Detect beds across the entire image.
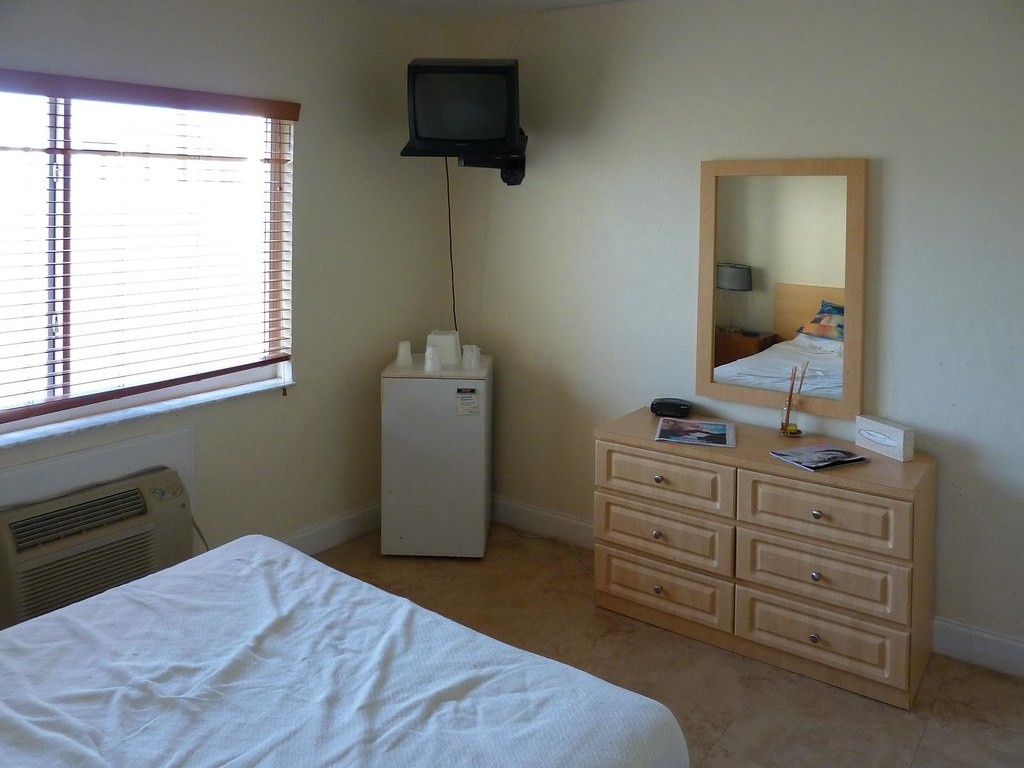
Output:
[0,534,694,768]
[714,282,845,401]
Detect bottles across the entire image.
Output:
[780,397,798,434]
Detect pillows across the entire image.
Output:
[789,333,844,353]
[796,299,844,342]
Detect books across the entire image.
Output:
[655,417,735,447]
[768,443,871,472]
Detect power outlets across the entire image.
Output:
[492,377,501,399]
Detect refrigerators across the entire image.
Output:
[378,353,494,558]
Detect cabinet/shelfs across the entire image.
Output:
[592,407,938,711]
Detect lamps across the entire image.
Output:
[717,263,753,332]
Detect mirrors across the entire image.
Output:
[695,158,869,419]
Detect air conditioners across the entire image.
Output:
[0,468,194,632]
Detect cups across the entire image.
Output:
[395,341,413,370]
[424,345,442,373]
[461,345,480,370]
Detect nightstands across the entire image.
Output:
[715,325,778,366]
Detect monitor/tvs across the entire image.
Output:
[407,57,519,153]
